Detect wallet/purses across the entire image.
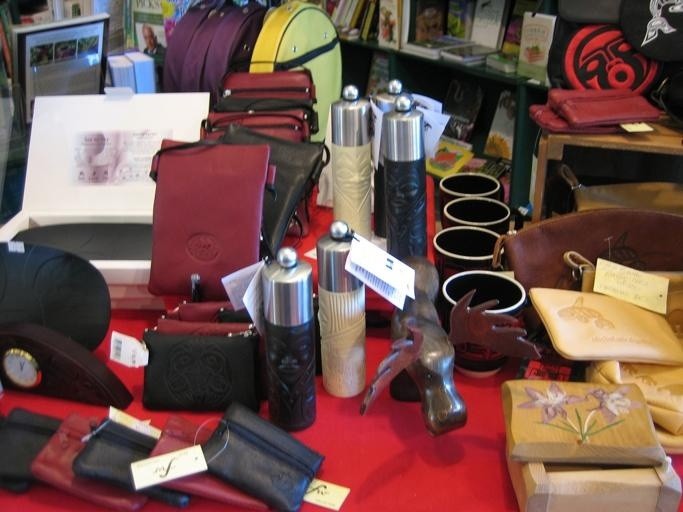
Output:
[30,413,148,510]
[142,304,264,414]
[0,405,63,493]
[0,404,322,511]
[72,415,191,508]
[202,404,324,512]
[528,90,662,136]
[148,416,270,511]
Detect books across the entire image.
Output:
[313,1,560,208]
[2,1,220,310]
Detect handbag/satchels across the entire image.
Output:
[528,287,683,368]
[501,379,666,466]
[492,210,683,296]
[564,251,683,336]
[585,361,683,434]
[145,70,326,297]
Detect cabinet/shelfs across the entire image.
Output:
[340,33,683,227]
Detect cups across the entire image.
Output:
[442,271,528,379]
[433,172,511,279]
